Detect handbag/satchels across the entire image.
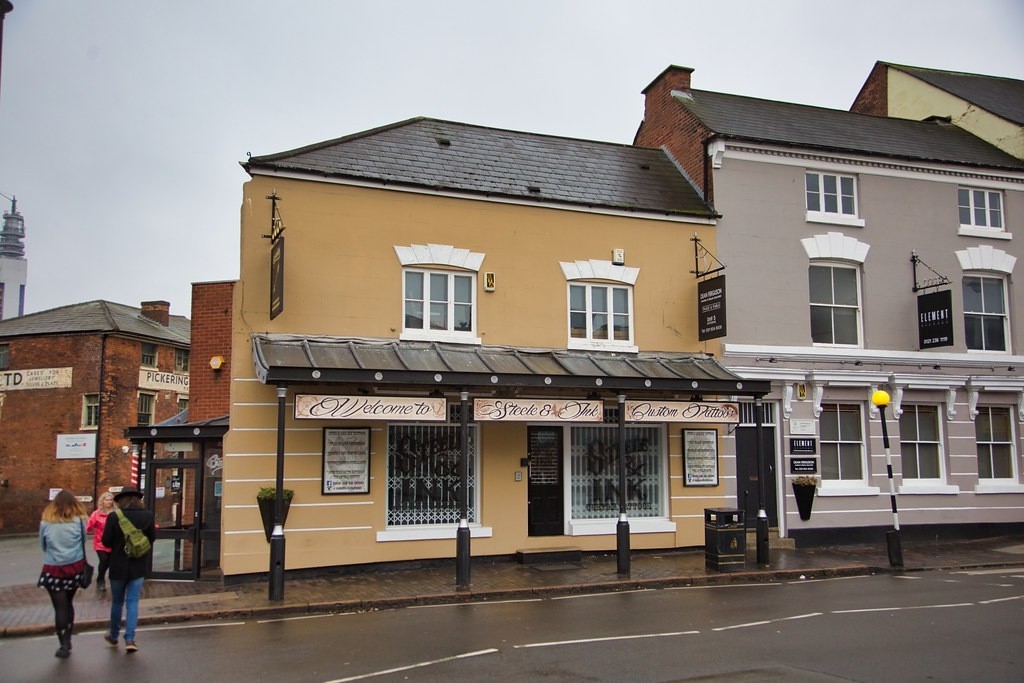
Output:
[79,562,94,588]
[116,509,152,558]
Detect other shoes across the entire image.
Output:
[97,579,106,591]
[56,648,68,657]
[67,640,71,649]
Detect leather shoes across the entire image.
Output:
[125,642,139,650]
[104,632,118,645]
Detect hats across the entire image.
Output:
[114,486,144,503]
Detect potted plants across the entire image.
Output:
[792,475,818,522]
[257,484,294,544]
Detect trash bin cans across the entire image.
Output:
[703,507,747,573]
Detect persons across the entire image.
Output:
[84,492,120,592]
[101,485,158,654]
[36,489,95,659]
